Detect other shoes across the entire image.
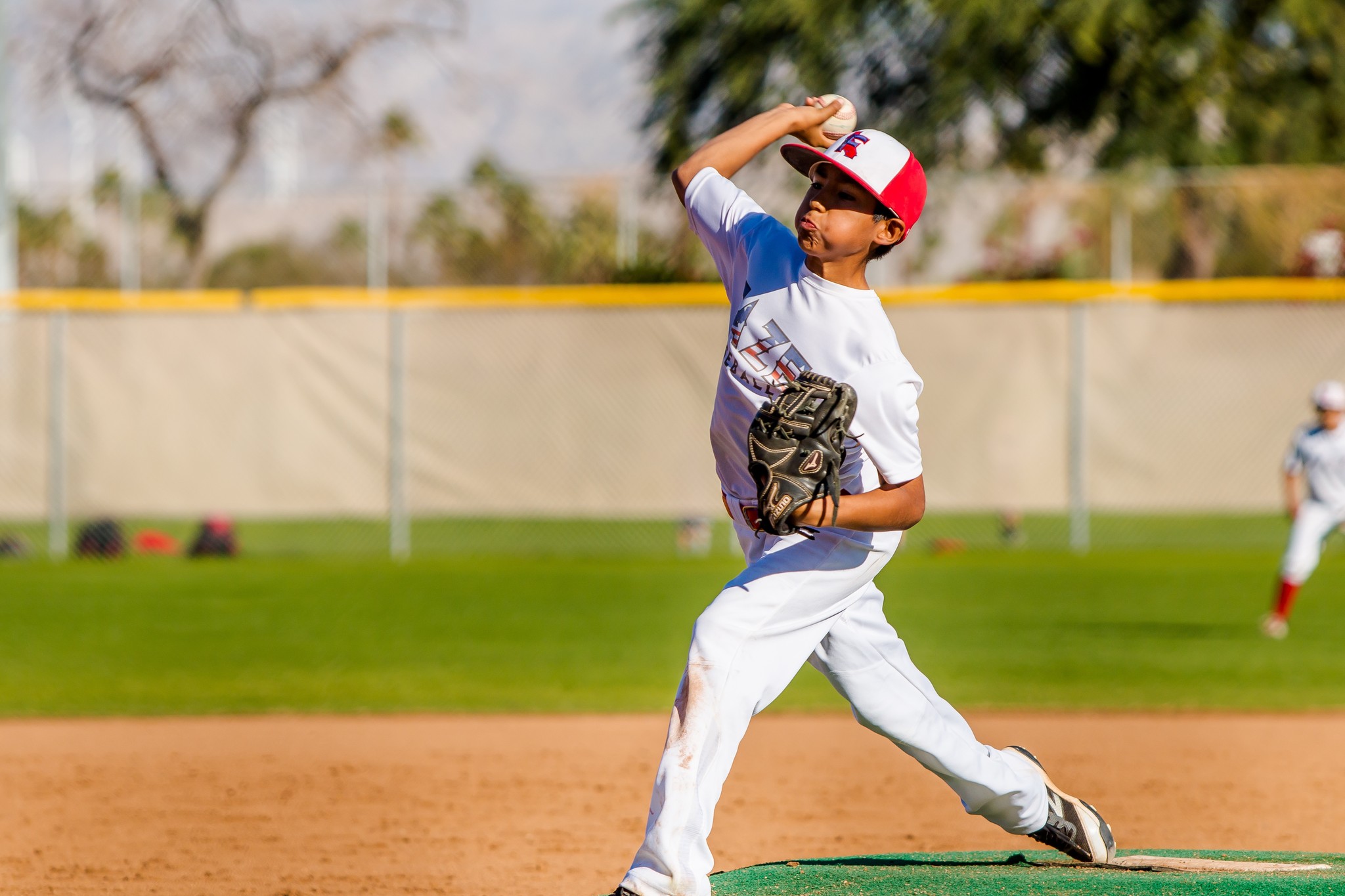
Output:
[1259,611,1291,641]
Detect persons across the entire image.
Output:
[1261,380,1345,640]
[607,99,1119,896]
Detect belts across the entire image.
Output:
[721,486,862,533]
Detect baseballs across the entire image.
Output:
[811,91,856,138]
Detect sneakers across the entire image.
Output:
[998,744,1117,865]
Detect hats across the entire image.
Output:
[778,128,928,246]
[1309,378,1345,413]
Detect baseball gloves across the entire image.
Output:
[747,369,857,540]
